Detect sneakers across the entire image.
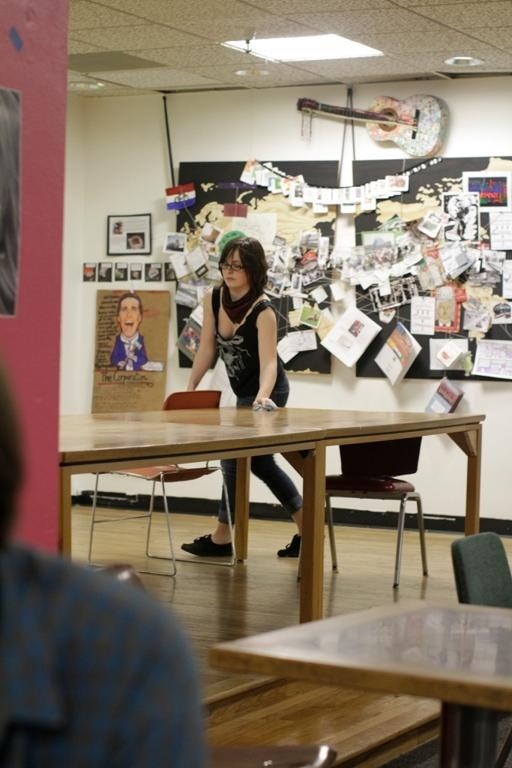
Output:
[278,533,301,558]
[182,535,233,557]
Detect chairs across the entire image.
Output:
[87,390,237,577]
[297,436,429,588]
[451,531,512,609]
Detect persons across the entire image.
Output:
[0,363,211,768]
[181,237,327,558]
[110,293,147,371]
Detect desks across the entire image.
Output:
[209,603,512,767]
[59,407,487,624]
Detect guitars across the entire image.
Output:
[298,95,449,159]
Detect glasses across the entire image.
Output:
[221,261,246,272]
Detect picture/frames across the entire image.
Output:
[463,169,511,212]
